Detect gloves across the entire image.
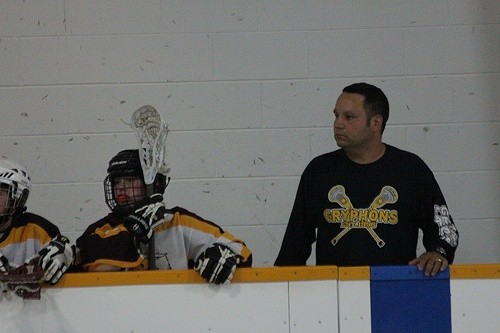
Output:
[194,243,240,285]
[26,235,75,284]
[0,253,10,275]
[126,192,174,243]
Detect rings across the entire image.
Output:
[436,259,442,263]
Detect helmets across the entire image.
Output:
[107,149,166,194]
[0,167,31,205]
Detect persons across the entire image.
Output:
[274,83,459,277]
[76,148,252,285]
[0,167,76,286]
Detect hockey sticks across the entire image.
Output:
[120,103,171,271]
